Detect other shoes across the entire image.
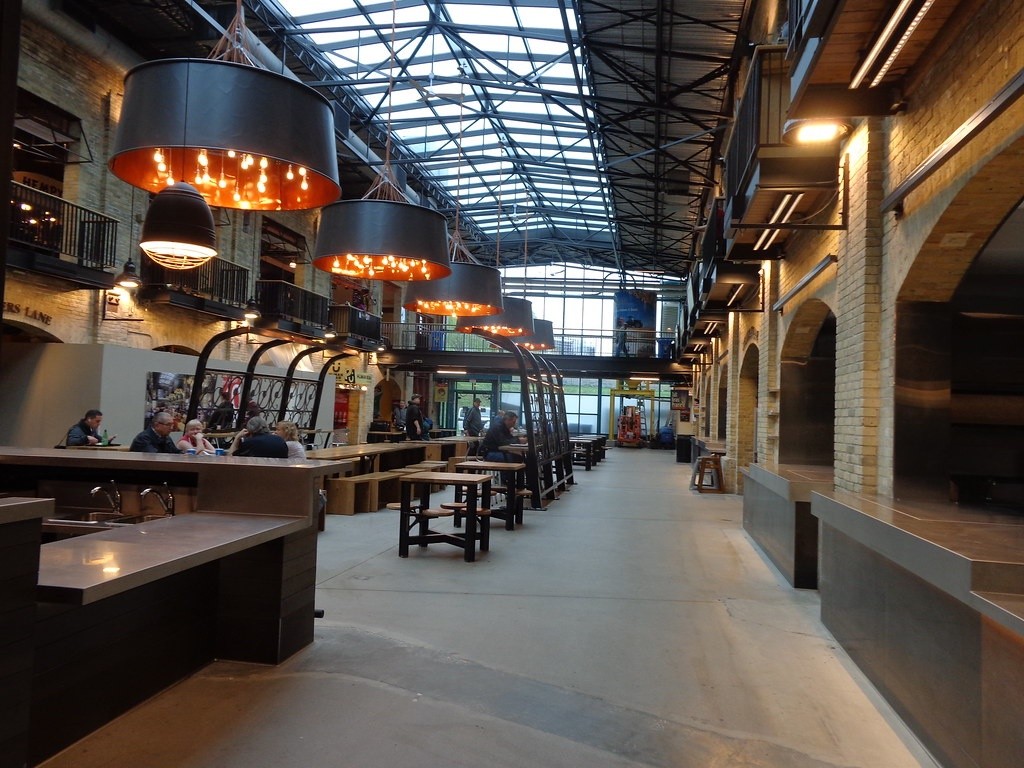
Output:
[515,487,532,496]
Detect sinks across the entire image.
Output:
[113,513,174,529]
[46,509,121,526]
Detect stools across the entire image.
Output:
[690,456,724,494]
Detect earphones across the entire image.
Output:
[88,418,90,421]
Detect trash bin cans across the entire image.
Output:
[676,435,695,463]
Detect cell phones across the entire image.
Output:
[112,435,116,438]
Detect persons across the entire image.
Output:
[406,394,433,441]
[275,419,307,459]
[463,398,483,462]
[352,287,369,310]
[207,391,234,430]
[392,400,407,432]
[65,408,112,446]
[175,418,216,454]
[227,416,289,459]
[480,407,527,491]
[245,401,261,428]
[129,411,184,454]
[615,322,630,357]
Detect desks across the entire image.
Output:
[203,428,607,563]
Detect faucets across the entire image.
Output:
[138,477,175,514]
[84,476,124,515]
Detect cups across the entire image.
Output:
[187,448,196,455]
[215,449,224,456]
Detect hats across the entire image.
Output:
[474,398,481,402]
[412,394,422,400]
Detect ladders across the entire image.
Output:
[637,398,648,443]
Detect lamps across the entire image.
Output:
[455,141,535,338]
[240,211,262,319]
[107,0,341,212]
[114,185,143,288]
[403,84,504,317]
[313,0,452,282]
[323,276,339,338]
[782,118,855,146]
[377,301,387,350]
[489,187,555,351]
[138,0,219,270]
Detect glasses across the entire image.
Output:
[157,421,174,427]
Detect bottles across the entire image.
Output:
[102,429,108,446]
